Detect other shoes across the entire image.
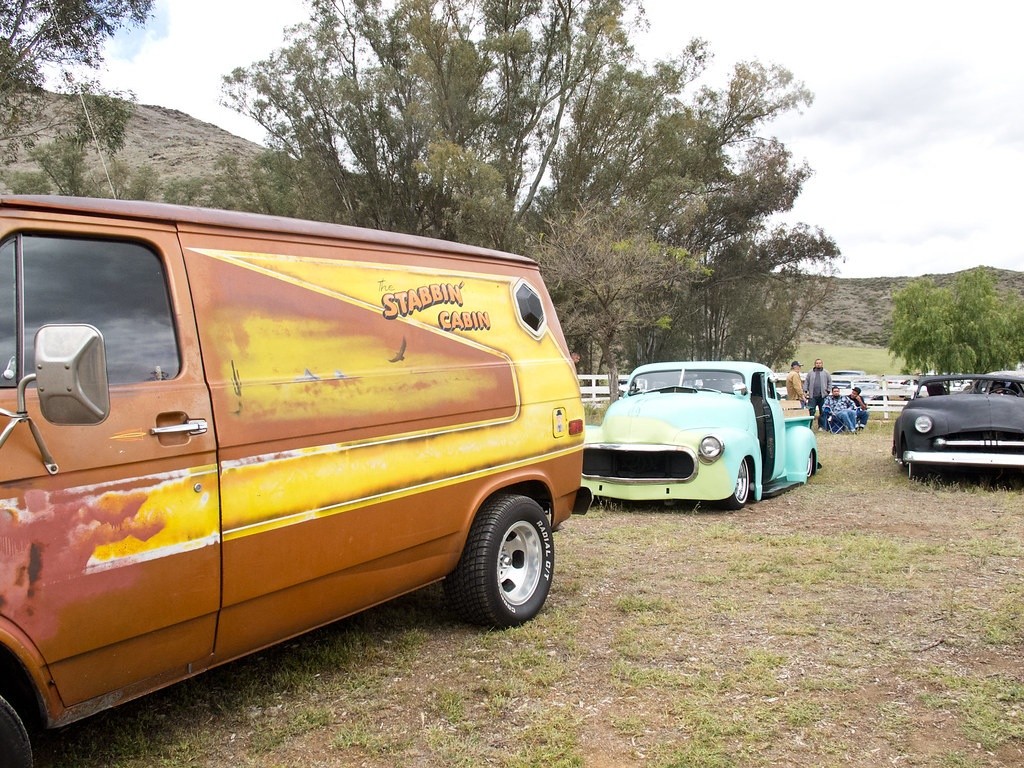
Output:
[817,428,824,432]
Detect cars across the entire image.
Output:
[580,360,822,512]
[829,370,866,389]
[857,385,889,407]
[892,374,1024,481]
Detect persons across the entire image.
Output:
[571,352,580,365]
[786,361,806,405]
[848,387,869,430]
[803,359,831,431]
[822,387,857,433]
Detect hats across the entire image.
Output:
[852,387,862,394]
[791,361,803,367]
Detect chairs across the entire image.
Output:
[703,380,733,393]
[824,407,848,434]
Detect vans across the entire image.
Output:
[0,194,593,768]
